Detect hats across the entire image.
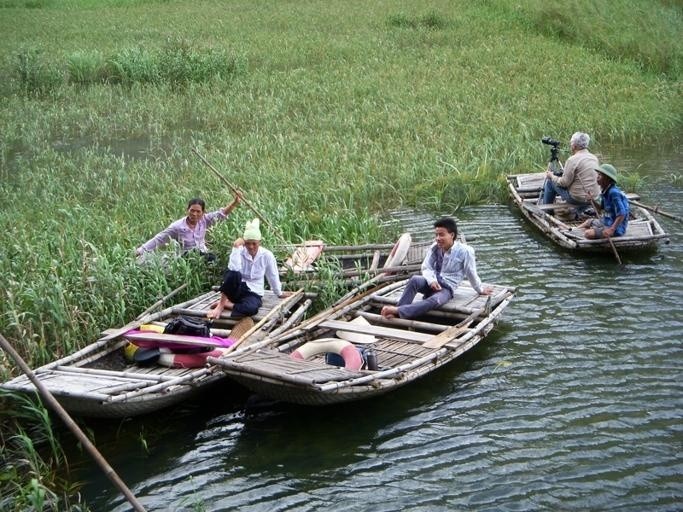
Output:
[242,219,261,241]
[335,314,380,343]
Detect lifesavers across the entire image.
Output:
[289,338,364,371]
[124,325,165,365]
[383,233,412,276]
[157,346,228,368]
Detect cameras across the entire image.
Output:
[540,135,563,151]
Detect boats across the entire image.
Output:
[207,275,517,403]
[1,279,311,424]
[504,170,668,255]
[275,235,468,286]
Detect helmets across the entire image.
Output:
[594,164,618,184]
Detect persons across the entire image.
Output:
[381,217,492,321]
[129,189,241,264]
[542,130,600,217]
[577,165,629,240]
[205,218,289,320]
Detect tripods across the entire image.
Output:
[535,156,565,206]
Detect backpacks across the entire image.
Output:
[162,315,215,353]
[325,344,370,370]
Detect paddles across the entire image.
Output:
[205,241,328,247]
[422,292,514,348]
[98,283,187,340]
[522,202,572,231]
[301,271,386,330]
